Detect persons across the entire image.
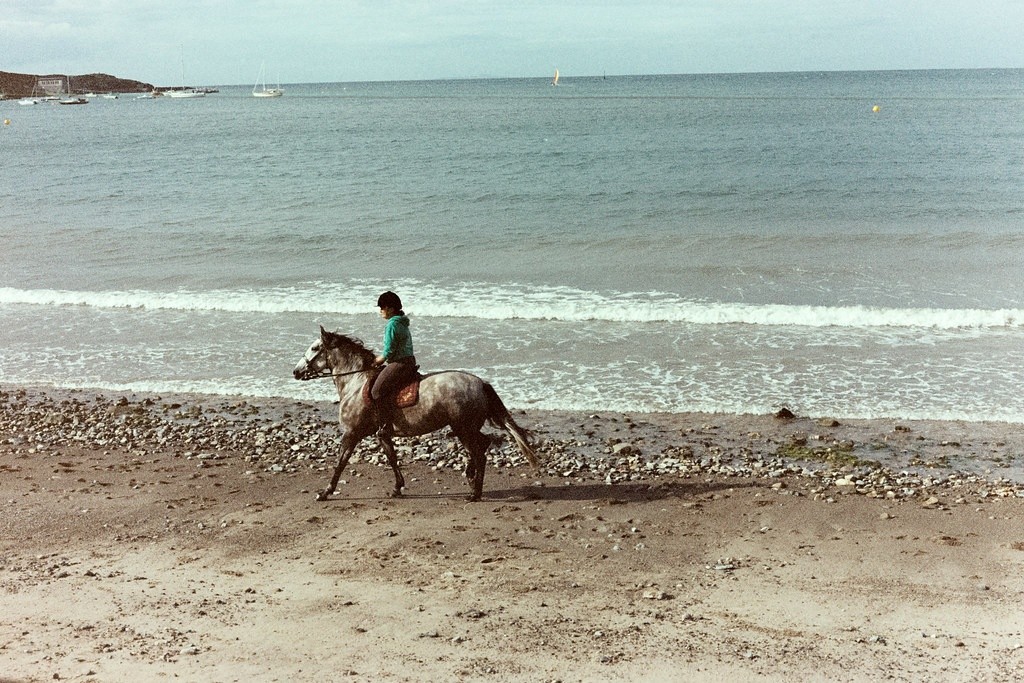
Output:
[368,290,416,436]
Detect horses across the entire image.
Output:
[292,326,540,501]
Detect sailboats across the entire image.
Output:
[251,60,286,99]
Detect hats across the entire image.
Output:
[373,291,402,310]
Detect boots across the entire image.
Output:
[373,395,395,437]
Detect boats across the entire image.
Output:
[104,94,118,100]
[59,98,91,105]
[138,86,221,97]
[18,95,61,107]
